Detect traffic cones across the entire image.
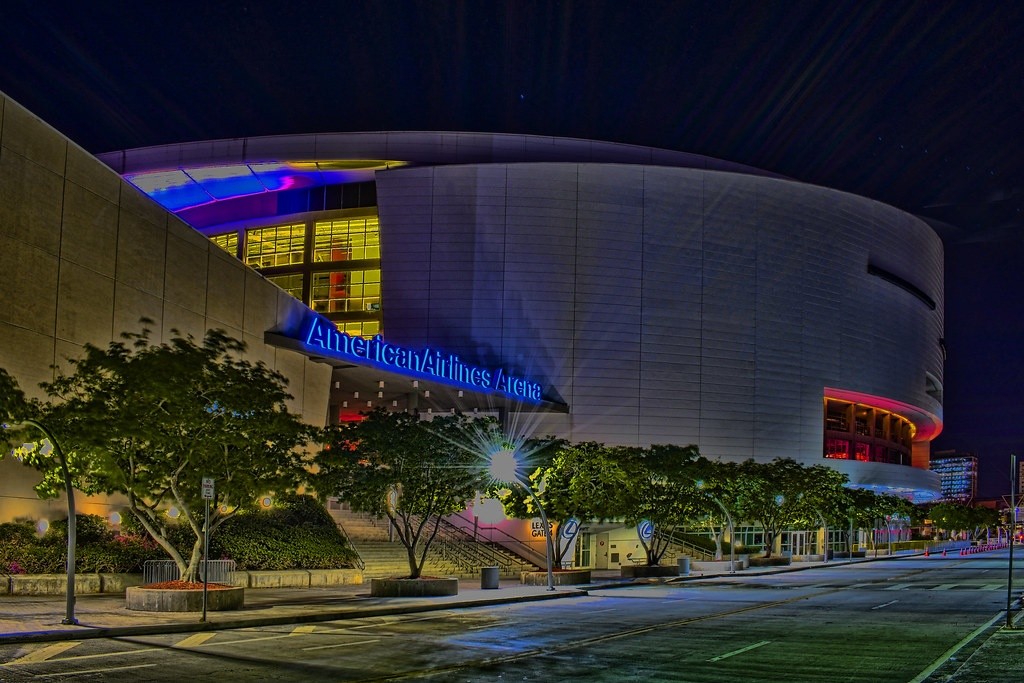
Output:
[925,546,930,556]
[960,542,1007,555]
[942,548,946,556]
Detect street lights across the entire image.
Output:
[0,419,78,624]
[800,493,828,563]
[492,458,555,591]
[698,482,736,573]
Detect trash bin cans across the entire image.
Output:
[827,550,833,559]
[677,559,689,576]
[739,554,750,567]
[860,548,866,555]
[893,543,899,551]
[481,568,499,589]
[782,551,792,562]
